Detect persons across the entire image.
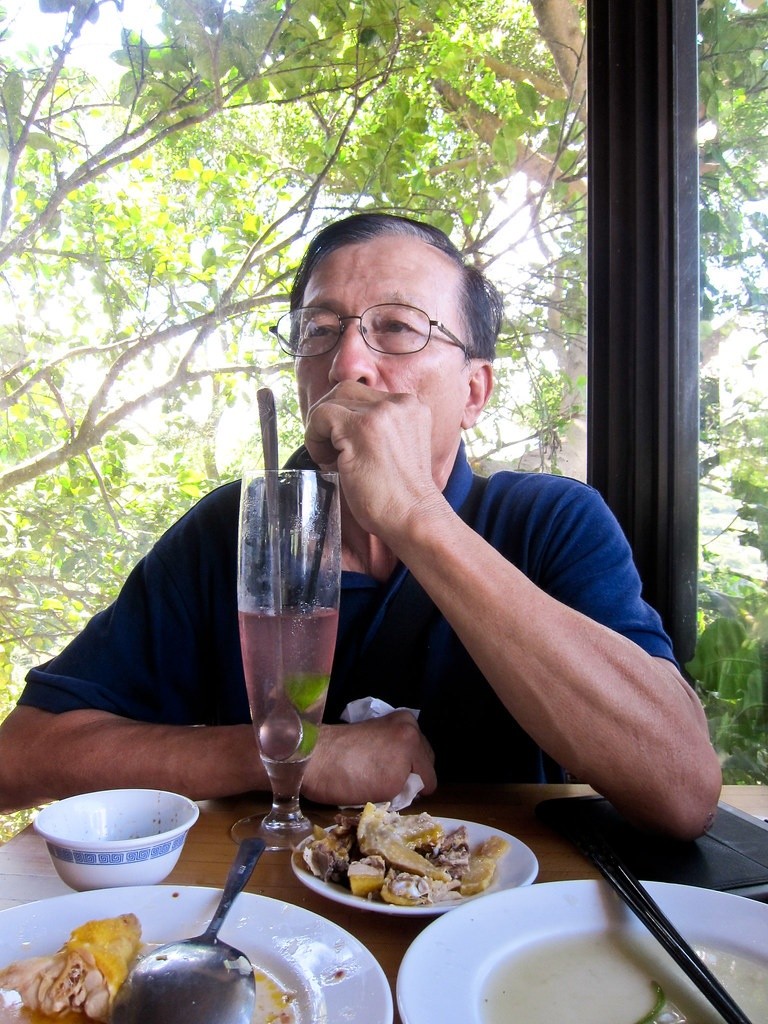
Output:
[0,206,721,843]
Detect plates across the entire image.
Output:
[289,810,541,916]
[2,883,392,1024]
[394,872,768,1023]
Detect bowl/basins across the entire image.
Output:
[32,788,199,895]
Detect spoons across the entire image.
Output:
[254,382,307,761]
[109,836,261,1024]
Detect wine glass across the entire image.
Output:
[230,466,343,850]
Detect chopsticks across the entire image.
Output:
[584,838,752,1023]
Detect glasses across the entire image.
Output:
[267,303,469,362]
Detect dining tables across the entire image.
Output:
[0,800,768,1024]
